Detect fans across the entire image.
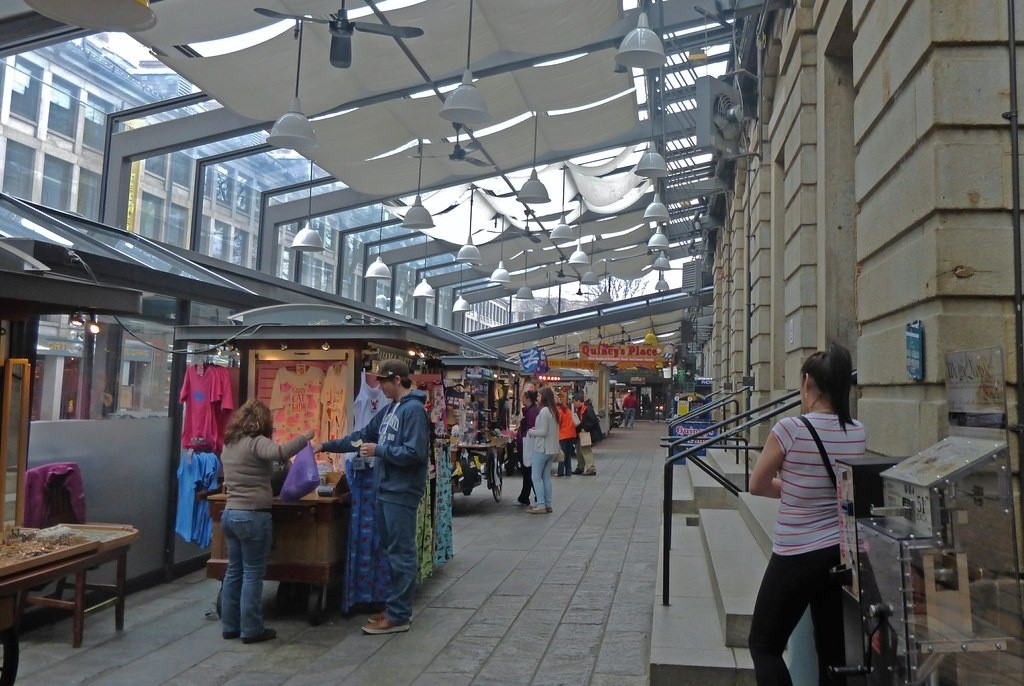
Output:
[252,0,426,70]
[487,209,551,243]
[408,123,494,168]
[695,75,743,156]
[551,257,577,280]
[569,273,591,296]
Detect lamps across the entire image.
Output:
[438,0,492,126]
[292,160,325,253]
[516,110,551,204]
[412,236,435,299]
[401,140,436,228]
[407,345,425,358]
[568,198,591,266]
[452,265,470,313]
[489,215,511,284]
[516,249,534,300]
[364,206,392,281]
[265,14,318,149]
[457,187,483,261]
[614,0,671,290]
[538,279,557,316]
[19,0,158,35]
[583,237,598,287]
[548,168,576,242]
[66,309,102,336]
[596,259,613,304]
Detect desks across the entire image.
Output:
[204,491,352,627]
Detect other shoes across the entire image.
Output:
[571,469,584,474]
[242,628,277,644]
[564,473,570,476]
[513,499,526,506]
[547,507,553,513]
[222,631,239,639]
[582,470,596,476]
[527,507,546,513]
[555,474,564,476]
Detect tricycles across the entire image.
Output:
[437,356,520,502]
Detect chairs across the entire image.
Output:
[24,460,131,649]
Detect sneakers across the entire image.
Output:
[367,611,412,625]
[361,616,410,633]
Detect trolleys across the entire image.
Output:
[196,471,353,626]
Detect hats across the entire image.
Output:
[628,389,632,392]
[366,359,409,378]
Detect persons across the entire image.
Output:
[517,390,539,505]
[622,391,639,429]
[555,395,577,477]
[748,341,866,686]
[222,398,314,644]
[572,395,603,476]
[526,387,559,514]
[642,394,650,415]
[311,359,430,633]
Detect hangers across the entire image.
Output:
[186,446,212,455]
[191,353,236,369]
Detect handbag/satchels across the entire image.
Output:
[579,429,591,446]
[557,445,565,462]
[572,413,581,427]
[522,430,534,466]
[280,440,321,502]
